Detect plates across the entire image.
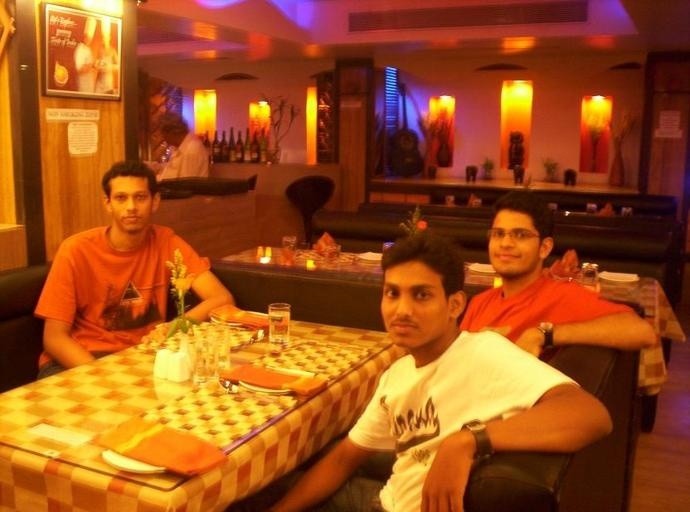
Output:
[356,249,385,263]
[599,269,641,285]
[467,261,495,274]
[211,310,269,325]
[239,363,316,393]
[102,448,167,475]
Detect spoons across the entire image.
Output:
[219,377,281,398]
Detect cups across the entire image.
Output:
[471,198,482,207]
[622,206,632,216]
[323,242,342,269]
[445,195,455,205]
[586,204,596,214]
[282,235,297,263]
[269,302,290,345]
[382,242,394,252]
[185,324,232,384]
[549,203,558,212]
[580,262,599,287]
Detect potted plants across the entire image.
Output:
[609,110,635,186]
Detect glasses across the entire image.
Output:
[487,228,541,243]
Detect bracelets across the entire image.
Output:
[458,418,494,460]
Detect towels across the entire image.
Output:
[88,414,228,477]
[218,363,328,399]
[209,303,290,333]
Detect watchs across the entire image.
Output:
[536,319,555,351]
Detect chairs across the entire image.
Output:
[283,176,335,246]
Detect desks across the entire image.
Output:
[224,244,687,433]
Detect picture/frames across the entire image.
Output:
[41,1,124,103]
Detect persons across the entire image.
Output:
[455,193,658,362]
[242,234,615,512]
[155,111,211,183]
[35,159,236,387]
[395,125,424,178]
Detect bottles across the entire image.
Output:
[207,127,267,163]
[152,348,191,384]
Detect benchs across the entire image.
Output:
[464,299,645,512]
[0,260,53,392]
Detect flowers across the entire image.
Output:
[397,204,431,250]
[163,248,201,338]
